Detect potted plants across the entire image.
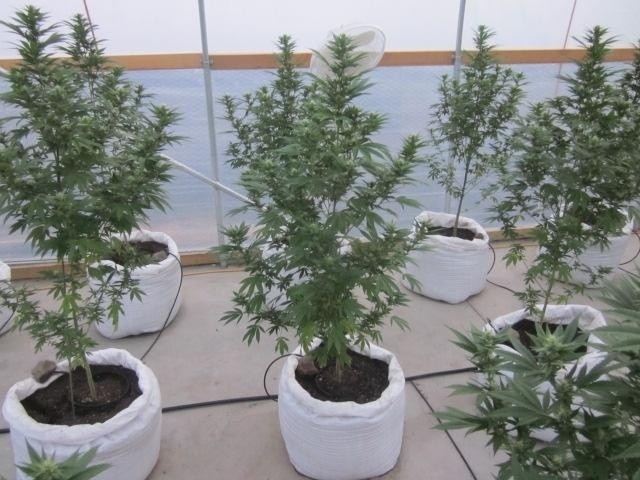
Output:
[209,29,447,479]
[13,437,111,479]
[545,28,638,289]
[258,32,354,322]
[0,3,163,480]
[85,70,183,338]
[476,25,640,447]
[401,25,532,306]
[433,268,639,479]
